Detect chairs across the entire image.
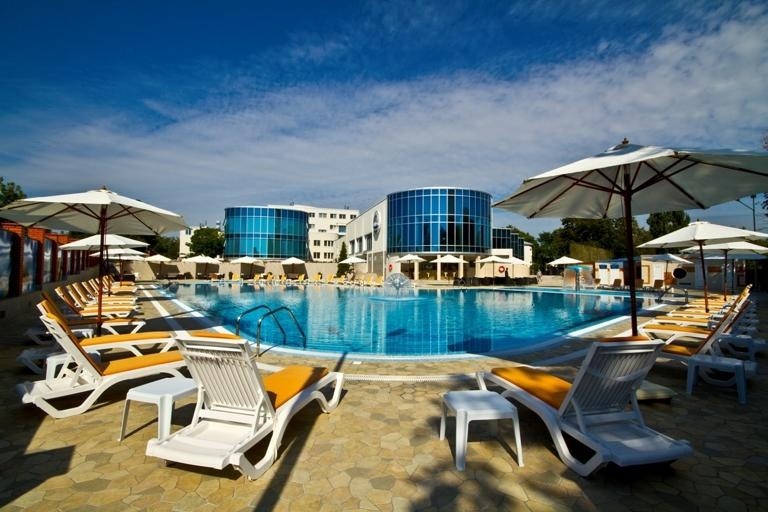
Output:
[13,275,197,419]
[146,331,345,481]
[599,283,767,405]
[413,269,455,280]
[454,276,536,286]
[585,278,664,293]
[165,271,383,287]
[476,336,694,477]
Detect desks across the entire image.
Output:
[439,390,525,472]
[117,377,201,466]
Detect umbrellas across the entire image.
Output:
[3,187,187,337]
[490,138,768,337]
[635,219,766,314]
[679,239,766,302]
